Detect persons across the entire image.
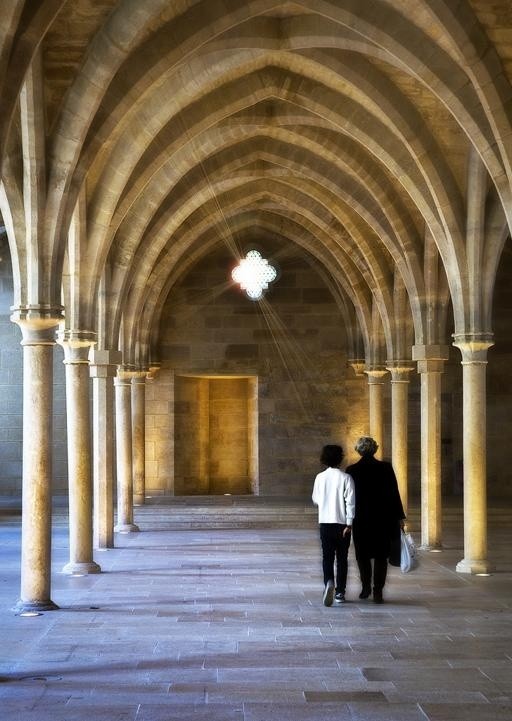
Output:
[343,436,407,606]
[310,443,358,608]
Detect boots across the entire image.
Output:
[358,574,373,600]
[373,586,386,604]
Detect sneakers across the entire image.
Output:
[320,579,335,608]
[335,593,346,603]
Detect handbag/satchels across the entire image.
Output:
[388,527,420,575]
